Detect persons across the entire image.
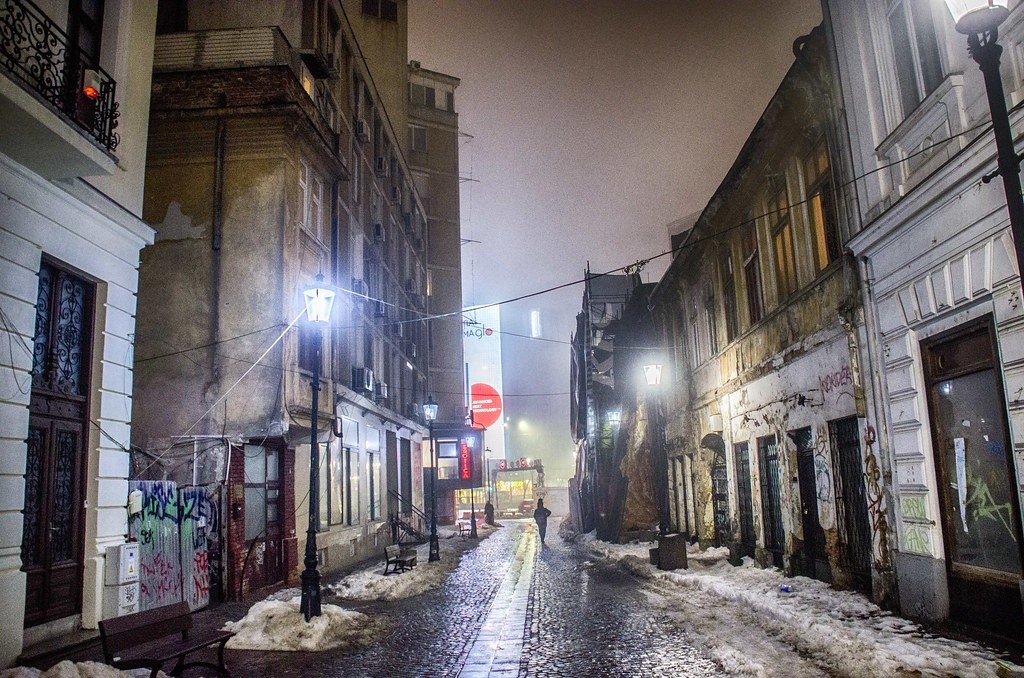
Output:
[484,501,495,526]
[534,498,552,543]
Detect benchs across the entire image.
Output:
[459,523,470,537]
[384,544,416,576]
[98,600,237,678]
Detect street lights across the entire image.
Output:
[485,447,491,502]
[642,359,669,535]
[943,0,1024,295]
[299,273,338,624]
[422,394,441,563]
[464,434,478,538]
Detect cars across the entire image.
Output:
[519,499,534,512]
